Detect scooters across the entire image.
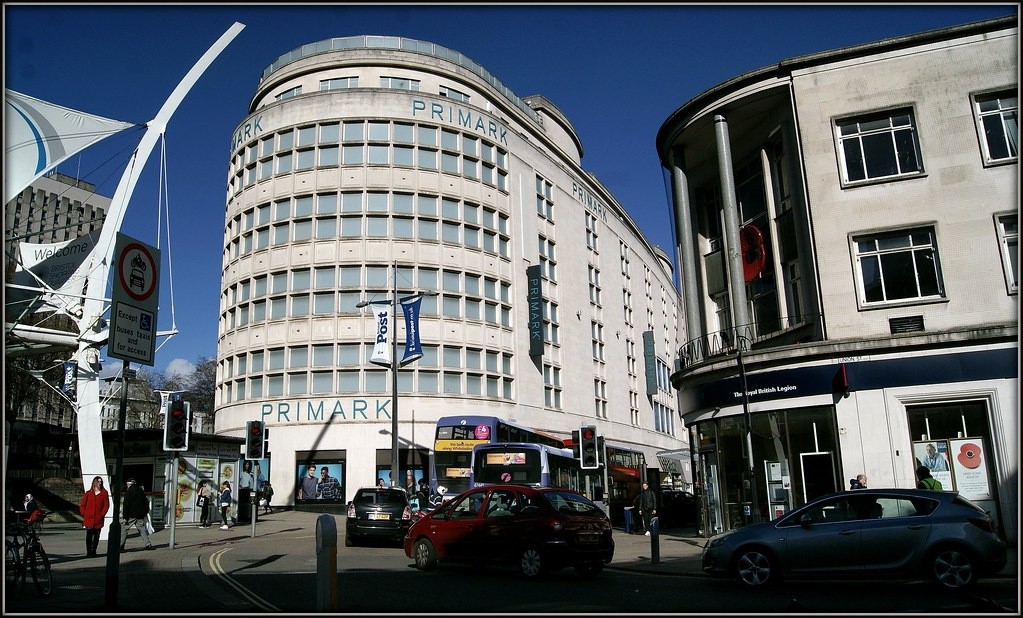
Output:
[407,503,466,529]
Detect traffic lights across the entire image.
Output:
[245,420,266,461]
[572,430,580,458]
[163,399,190,452]
[580,425,599,469]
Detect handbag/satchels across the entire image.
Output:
[408,494,420,512]
[196,488,206,507]
[259,498,267,507]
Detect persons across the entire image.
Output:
[639,481,656,537]
[218,480,232,530]
[916,467,942,490]
[80,476,109,558]
[403,470,416,494]
[196,479,211,528]
[298,464,321,500]
[316,467,341,499]
[120,478,151,551]
[923,444,946,472]
[24,494,38,520]
[378,478,384,487]
[850,474,867,490]
[239,459,265,487]
[262,482,274,515]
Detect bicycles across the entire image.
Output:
[5,506,56,598]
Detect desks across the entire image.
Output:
[12,510,29,533]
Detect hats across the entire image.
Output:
[126,478,137,482]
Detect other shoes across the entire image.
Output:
[645,531,650,536]
[86,551,94,558]
[92,552,99,558]
[119,544,125,552]
[198,523,210,528]
[269,512,273,514]
[219,524,228,530]
[145,544,153,550]
[263,512,267,515]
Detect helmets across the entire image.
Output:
[436,486,448,496]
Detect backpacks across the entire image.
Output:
[264,484,274,498]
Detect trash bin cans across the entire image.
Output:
[237,489,259,521]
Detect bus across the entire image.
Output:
[428,415,563,511]
[470,442,616,521]
[563,439,648,521]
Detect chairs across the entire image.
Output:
[26,510,52,536]
[487,495,510,516]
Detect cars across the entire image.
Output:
[699,488,1007,595]
[662,490,696,524]
[403,484,615,582]
[345,487,418,549]
